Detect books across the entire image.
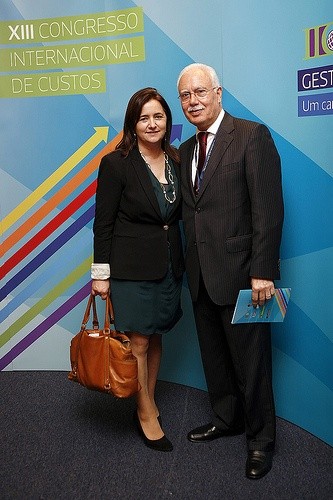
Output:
[232,288,292,324]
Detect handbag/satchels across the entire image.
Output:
[67,292,141,399]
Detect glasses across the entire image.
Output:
[177,86,220,104]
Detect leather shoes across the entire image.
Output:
[187,422,246,442]
[246,447,275,480]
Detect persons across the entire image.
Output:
[175,63,284,481]
[91,87,183,452]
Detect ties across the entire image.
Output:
[193,132,209,198]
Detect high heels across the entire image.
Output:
[156,411,163,426]
[134,409,173,452]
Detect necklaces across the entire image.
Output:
[138,150,176,204]
